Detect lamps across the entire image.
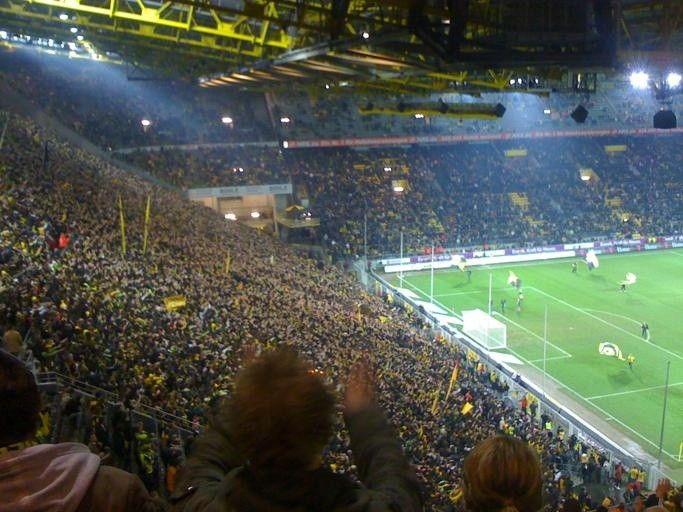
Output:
[510,74,541,89]
[573,72,589,91]
[613,71,681,92]
[448,80,468,89]
